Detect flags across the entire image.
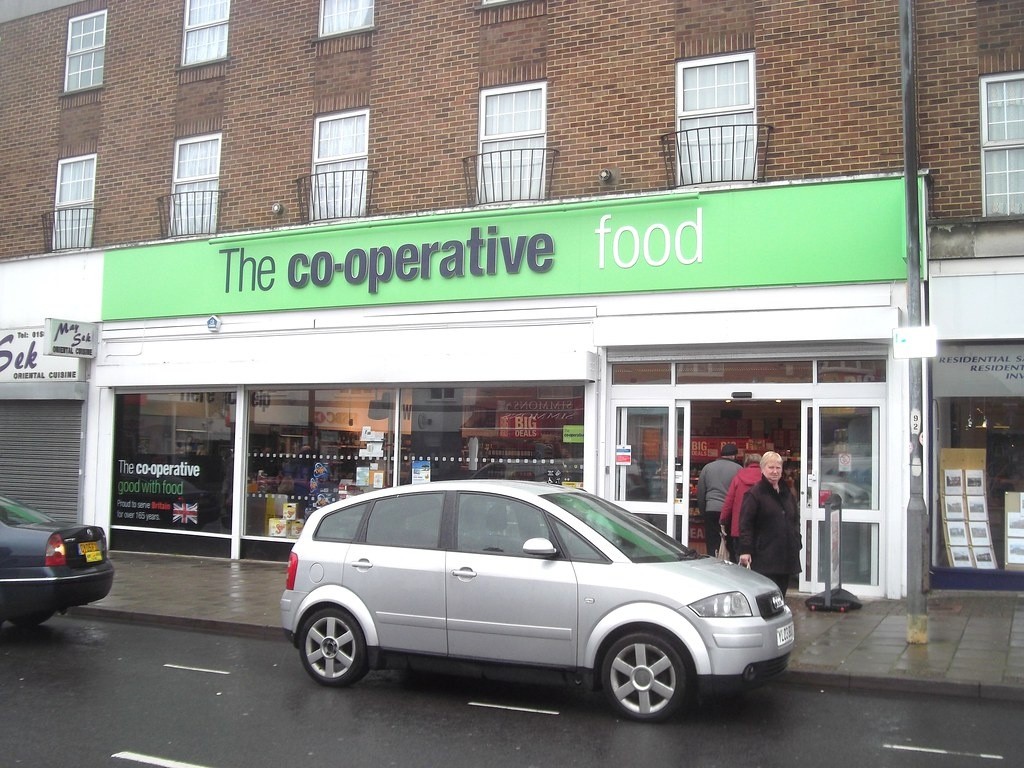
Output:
[173,502,198,525]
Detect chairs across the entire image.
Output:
[459,497,518,555]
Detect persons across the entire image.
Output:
[719,452,763,566]
[783,460,793,478]
[220,450,294,515]
[618,457,653,547]
[181,435,208,456]
[697,443,743,558]
[301,443,318,464]
[736,450,802,600]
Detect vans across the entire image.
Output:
[471,460,560,482]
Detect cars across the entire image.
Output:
[0,496,115,627]
[118,470,222,531]
[280,479,795,723]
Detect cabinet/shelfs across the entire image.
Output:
[644,474,706,543]
[174,428,213,453]
[320,446,411,464]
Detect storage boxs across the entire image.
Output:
[268,503,305,537]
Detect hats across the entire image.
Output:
[720,444,738,456]
